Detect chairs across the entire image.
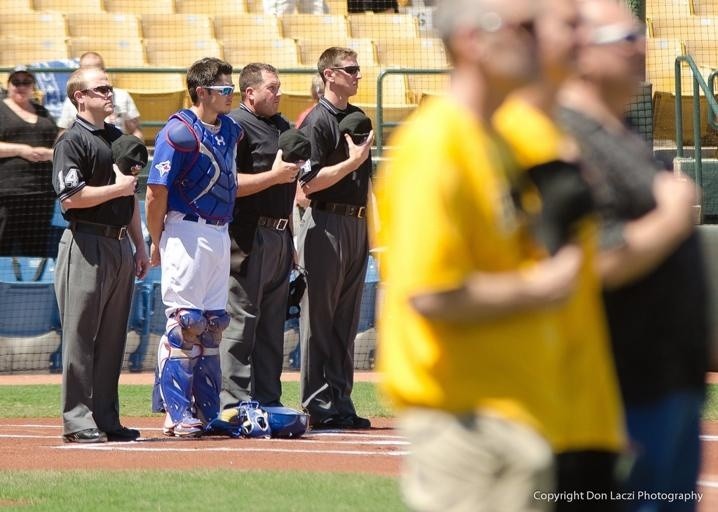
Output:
[645,0,718,146]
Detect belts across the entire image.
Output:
[258,216,289,232]
[309,201,367,219]
[183,215,226,227]
[68,221,129,242]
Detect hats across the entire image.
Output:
[513,158,592,259]
[338,111,373,157]
[111,134,148,177]
[8,65,37,84]
[278,128,310,165]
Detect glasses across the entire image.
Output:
[72,86,113,101]
[331,65,360,75]
[11,79,32,86]
[195,84,235,97]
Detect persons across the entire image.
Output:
[298,46,379,430]
[144,55,244,440]
[220,61,306,410]
[289,70,325,212]
[0,64,57,257]
[263,1,400,16]
[52,66,151,445]
[58,51,141,138]
[366,1,712,512]
